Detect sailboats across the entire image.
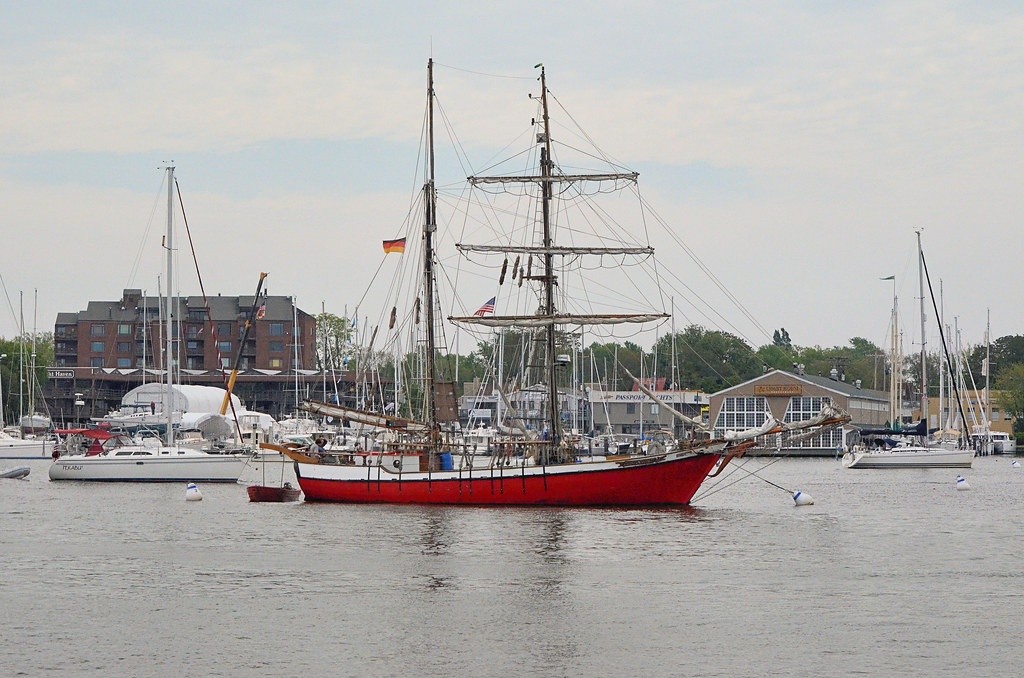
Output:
[259,58,853,507]
[840,229,992,469]
[0,160,267,482]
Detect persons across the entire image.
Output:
[310,438,327,459]
[386,438,399,452]
[150,401,155,415]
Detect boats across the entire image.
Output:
[242,447,303,502]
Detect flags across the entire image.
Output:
[473,296,495,317]
[383,237,406,254]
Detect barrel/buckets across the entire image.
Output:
[441,451,452,470]
